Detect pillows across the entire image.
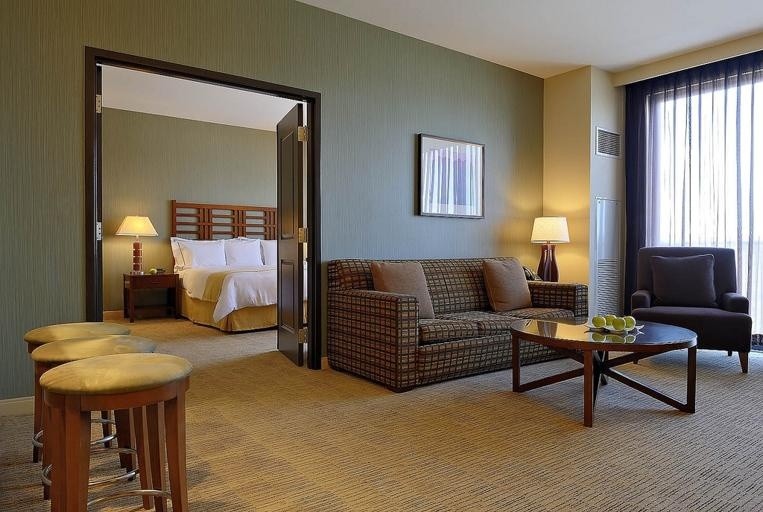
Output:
[482,259,533,313]
[171,236,277,274]
[369,262,436,319]
[649,253,720,308]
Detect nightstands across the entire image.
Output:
[122,272,179,323]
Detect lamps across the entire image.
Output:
[113,215,159,275]
[529,217,570,282]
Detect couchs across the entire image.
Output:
[326,256,589,393]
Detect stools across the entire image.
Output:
[22,320,194,511]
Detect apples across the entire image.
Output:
[592,333,636,344]
[149,269,158,275]
[591,314,636,330]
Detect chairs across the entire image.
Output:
[630,246,752,374]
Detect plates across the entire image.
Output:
[583,320,643,335]
[582,330,645,344]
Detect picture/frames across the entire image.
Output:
[414,133,485,220]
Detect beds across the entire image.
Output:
[170,200,307,335]
